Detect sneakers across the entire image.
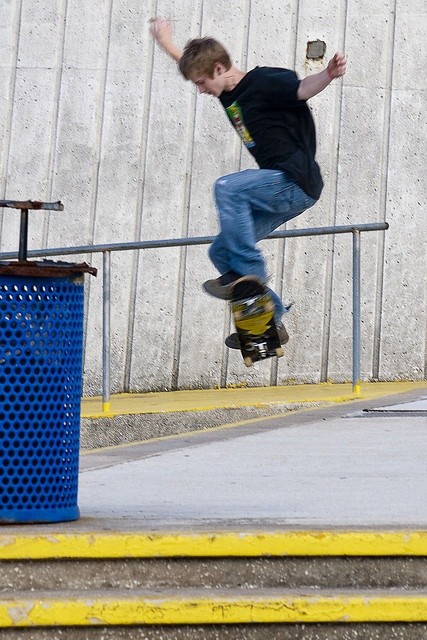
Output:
[225,323,289,348]
[202,270,264,300]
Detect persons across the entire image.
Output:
[138,15,347,350]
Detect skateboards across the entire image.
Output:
[229,274,283,367]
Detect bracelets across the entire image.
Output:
[325,67,336,81]
